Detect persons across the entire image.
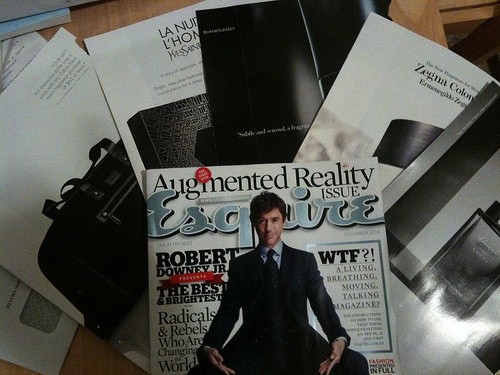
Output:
[185,190,369,375]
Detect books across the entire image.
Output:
[145,156,402,375]
[83,0,220,208]
[1,27,148,343]
[195,0,394,165]
[383,82,500,375]
[291,12,500,191]
[1,0,100,42]
